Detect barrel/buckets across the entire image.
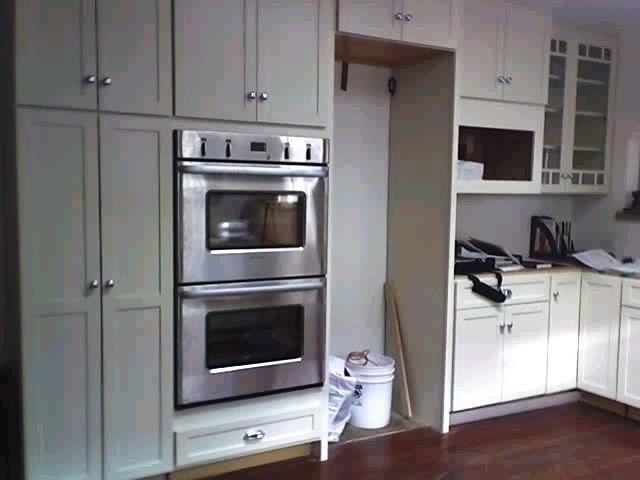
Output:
[345,357,396,429]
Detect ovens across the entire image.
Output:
[173,129,326,405]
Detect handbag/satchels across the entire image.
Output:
[455,255,505,303]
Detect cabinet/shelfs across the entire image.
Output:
[449,271,640,411]
[336,2,459,67]
[8,105,169,480]
[542,24,616,193]
[9,0,335,127]
[388,8,549,105]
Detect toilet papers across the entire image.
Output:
[624,139,640,193]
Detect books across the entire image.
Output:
[538,218,573,256]
